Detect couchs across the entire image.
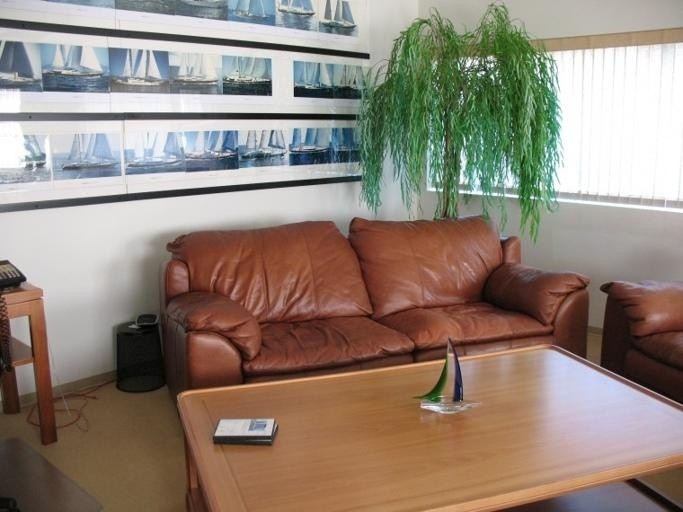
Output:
[160,235,590,418]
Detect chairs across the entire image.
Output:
[599,279,683,407]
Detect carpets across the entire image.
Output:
[0,439,105,512]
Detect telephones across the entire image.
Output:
[0,260,26,288]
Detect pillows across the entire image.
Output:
[169,220,373,325]
[349,215,504,319]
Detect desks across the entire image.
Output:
[0,282,57,445]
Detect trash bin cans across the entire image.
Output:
[116,321,165,392]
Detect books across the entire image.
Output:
[213,418,275,441]
[213,424,278,446]
